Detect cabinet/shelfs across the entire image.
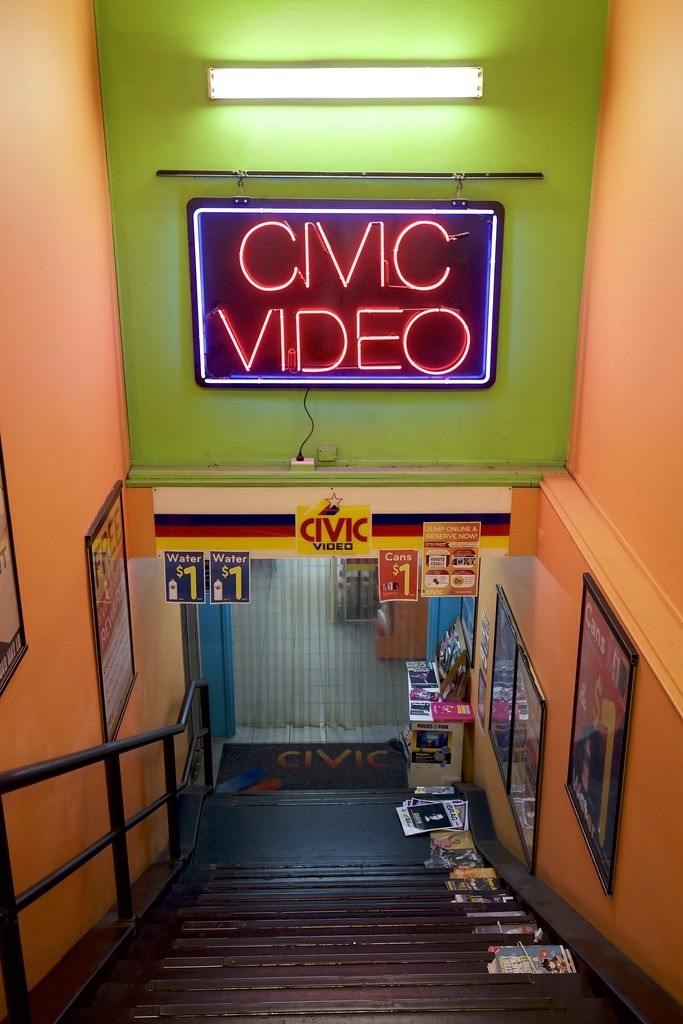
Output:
[375,566,428,659]
[407,660,465,789]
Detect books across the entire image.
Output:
[431,702,475,724]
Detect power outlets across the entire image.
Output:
[289,457,315,472]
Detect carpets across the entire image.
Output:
[215,738,411,792]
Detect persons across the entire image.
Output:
[414,810,445,825]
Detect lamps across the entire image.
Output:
[206,65,484,100]
